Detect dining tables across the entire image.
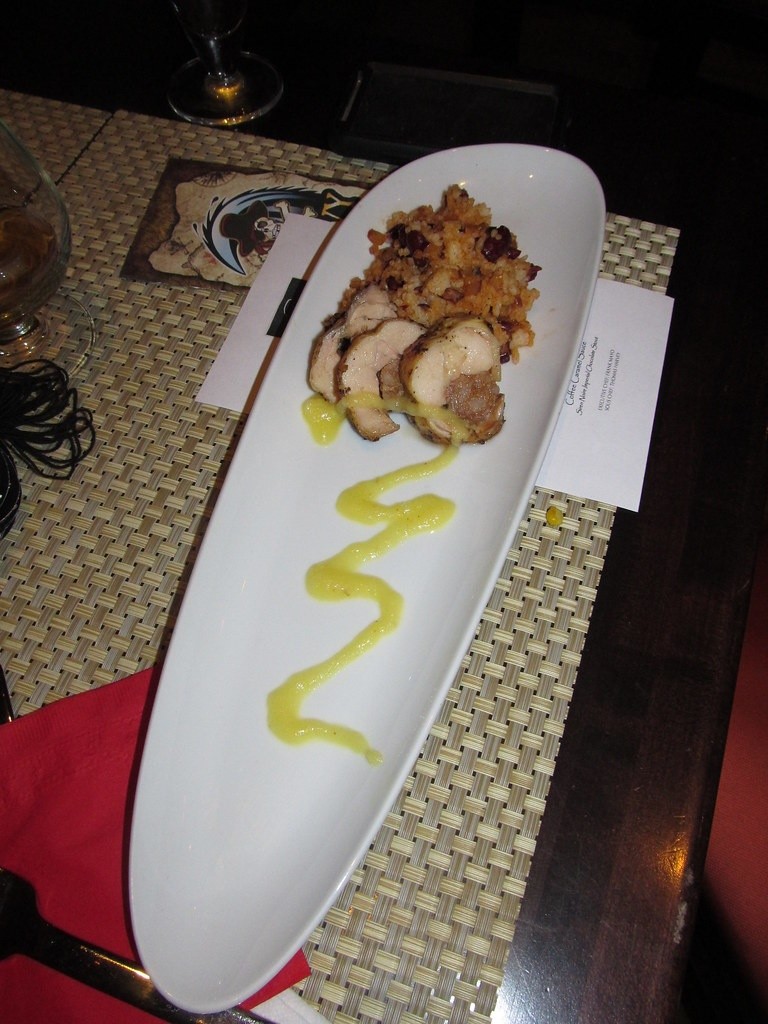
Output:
[0,14,768,1024]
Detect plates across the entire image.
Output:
[125,141,608,1013]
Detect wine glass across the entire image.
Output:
[0,121,97,382]
[164,0,285,126]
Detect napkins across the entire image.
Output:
[0,664,313,1024]
